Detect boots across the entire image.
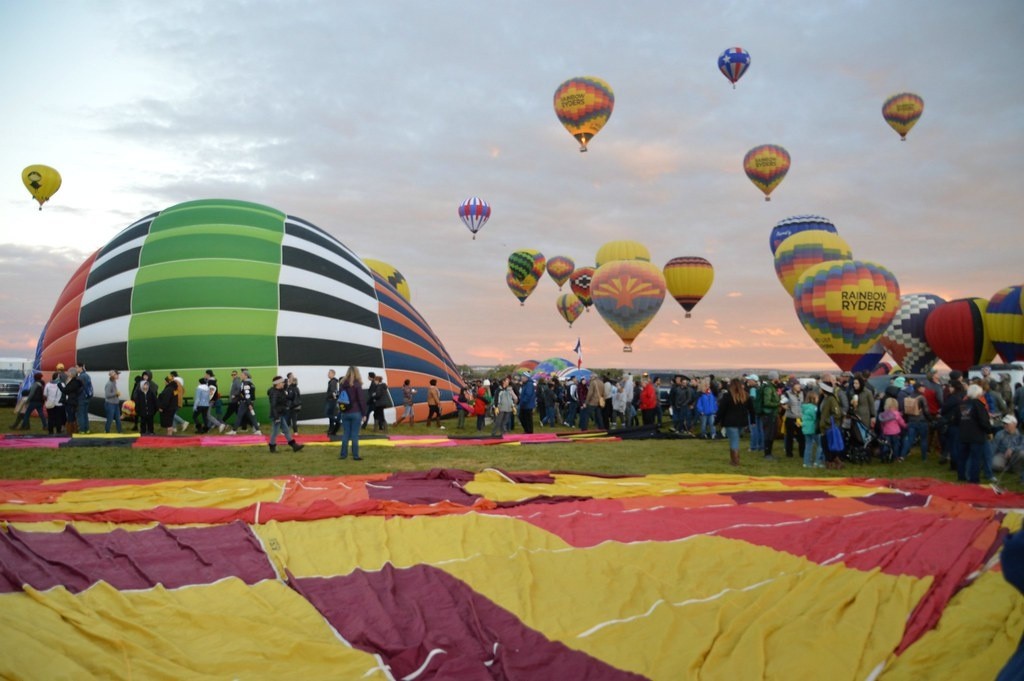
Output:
[288,439,304,452]
[728,447,741,467]
[268,443,279,453]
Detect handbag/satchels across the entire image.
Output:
[598,396,605,408]
[827,416,845,452]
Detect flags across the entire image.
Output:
[573,339,582,371]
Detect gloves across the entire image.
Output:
[494,407,500,416]
[512,406,518,416]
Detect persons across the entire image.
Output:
[9,354,1024,483]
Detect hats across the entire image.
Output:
[56,363,64,370]
[521,370,530,378]
[893,376,906,388]
[271,376,285,385]
[1000,413,1017,425]
[967,384,983,400]
[745,374,759,382]
[819,380,834,393]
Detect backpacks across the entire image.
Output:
[335,376,353,412]
[753,385,769,415]
[903,395,923,416]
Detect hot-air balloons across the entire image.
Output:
[506,269,540,306]
[42,199,502,438]
[927,296,999,382]
[589,259,667,354]
[510,359,599,388]
[557,291,583,328]
[507,248,546,297]
[546,254,575,291]
[792,260,901,374]
[594,241,648,265]
[458,196,491,240]
[21,164,62,211]
[662,255,716,318]
[568,267,595,312]
[359,258,410,305]
[881,90,925,142]
[987,284,1024,365]
[849,340,887,387]
[770,215,838,257]
[880,293,948,374]
[719,46,751,88]
[743,144,792,200]
[554,75,615,152]
[774,230,854,299]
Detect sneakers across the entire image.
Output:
[181,420,190,432]
[252,429,263,435]
[218,423,225,434]
[825,461,834,471]
[224,429,236,435]
[834,457,844,470]
